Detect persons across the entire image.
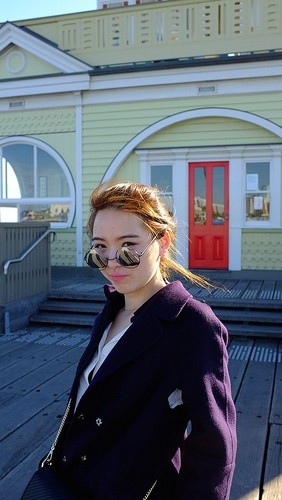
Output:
[21,181,237,499]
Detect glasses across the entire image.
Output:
[83,233,159,269]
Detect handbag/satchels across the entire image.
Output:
[21,461,79,500]
